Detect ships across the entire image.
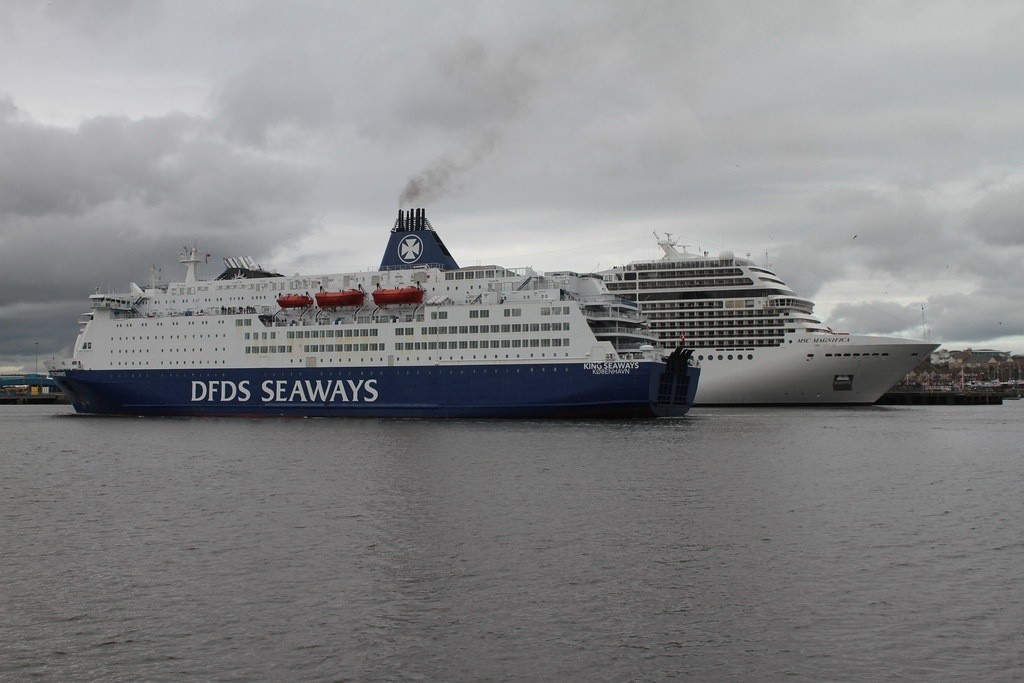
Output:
[591,230,940,405]
[48,207,701,417]
